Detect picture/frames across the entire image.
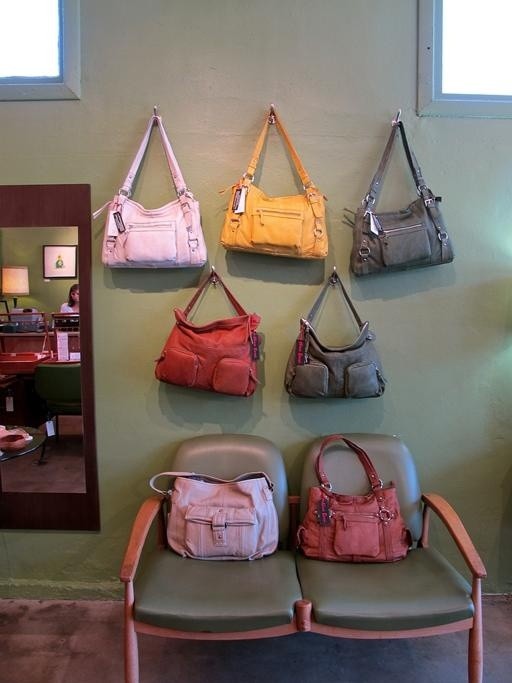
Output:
[45,243,76,280]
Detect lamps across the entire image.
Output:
[1,266,30,306]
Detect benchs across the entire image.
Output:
[116,432,489,683]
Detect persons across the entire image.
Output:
[59,285,80,314]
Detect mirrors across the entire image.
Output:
[0,182,102,533]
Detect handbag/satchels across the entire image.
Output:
[285,271,389,399]
[219,107,330,260]
[92,115,208,268]
[155,271,263,397]
[296,435,413,564]
[341,120,455,277]
[150,471,279,563]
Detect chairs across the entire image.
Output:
[32,363,85,465]
